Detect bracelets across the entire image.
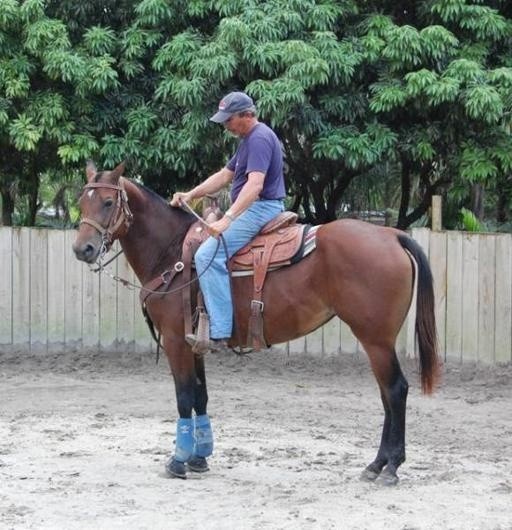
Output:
[225,211,235,221]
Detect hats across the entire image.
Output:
[209,92,253,122]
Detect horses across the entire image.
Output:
[69,157,439,487]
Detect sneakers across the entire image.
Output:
[185,334,230,356]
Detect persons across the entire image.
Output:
[170,91,287,355]
